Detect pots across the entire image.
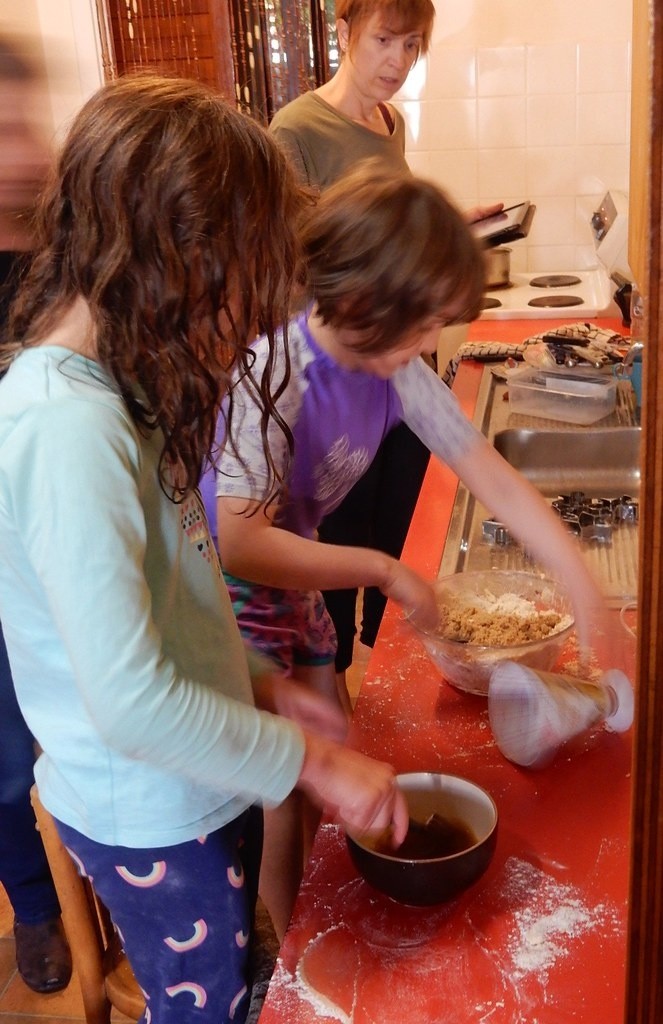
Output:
[484,244,512,287]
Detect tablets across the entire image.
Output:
[468,200,529,244]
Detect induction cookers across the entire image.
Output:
[449,189,629,318]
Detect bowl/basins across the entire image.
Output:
[344,769,499,907]
[403,569,576,696]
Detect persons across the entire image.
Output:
[0,40,412,1024]
[265,2,504,724]
[193,165,618,964]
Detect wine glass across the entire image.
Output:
[488,657,639,765]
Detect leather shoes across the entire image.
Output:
[13,915,73,994]
